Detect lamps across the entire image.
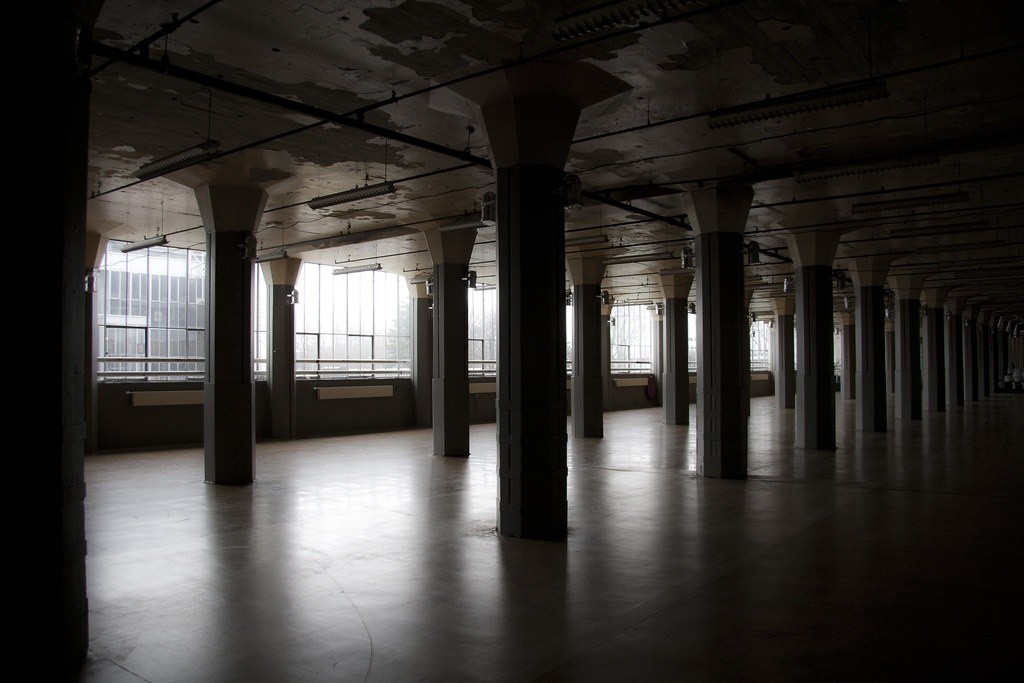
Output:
[477,191,497,226]
[459,263,477,289]
[133,89,222,180]
[750,311,757,322]
[120,201,170,253]
[306,138,395,209]
[425,278,434,296]
[332,246,383,276]
[684,303,696,314]
[680,248,695,268]
[740,240,761,264]
[832,271,846,289]
[655,302,664,314]
[554,174,587,207]
[596,290,610,307]
[437,188,491,234]
[783,275,796,294]
